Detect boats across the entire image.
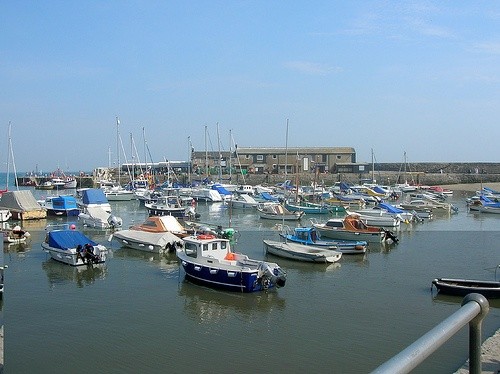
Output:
[174,233,289,293]
[78,206,122,229]
[465,177,500,213]
[112,213,238,257]
[262,206,400,264]
[429,263,500,298]
[37,194,81,217]
[0,264,8,293]
[35,176,78,190]
[40,223,107,266]
[76,187,112,214]
[4,225,30,243]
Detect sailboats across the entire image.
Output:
[97,117,455,220]
[0,121,48,222]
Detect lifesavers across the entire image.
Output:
[197,235,216,239]
